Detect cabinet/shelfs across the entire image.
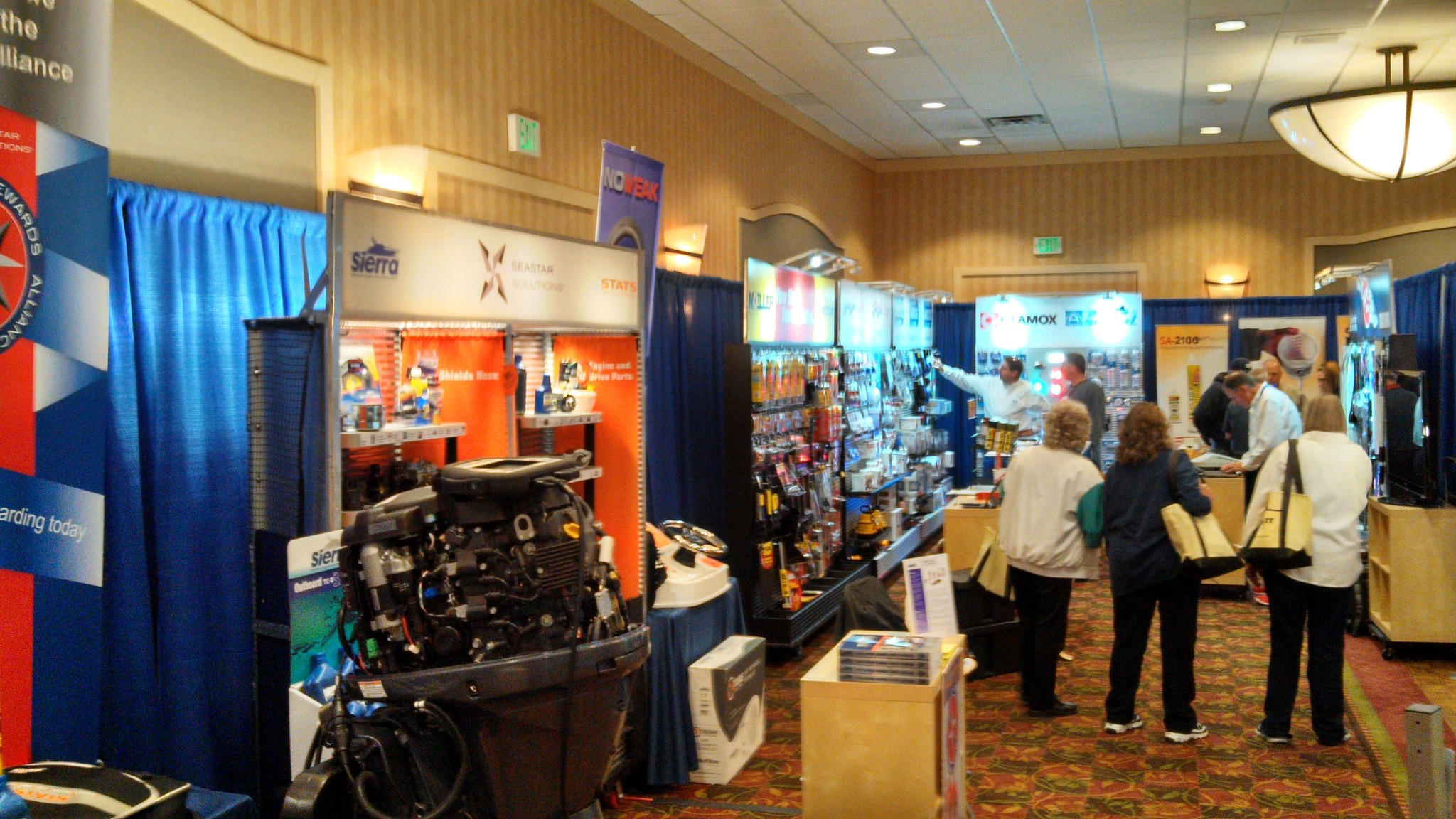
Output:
[1365,492,1456,645]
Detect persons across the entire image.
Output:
[995,399,1110,717]
[1098,403,1214,743]
[1188,353,1342,611]
[1233,395,1374,746]
[1058,351,1106,477]
[930,354,1051,483]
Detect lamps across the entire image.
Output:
[1264,43,1456,181]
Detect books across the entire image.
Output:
[839,632,943,687]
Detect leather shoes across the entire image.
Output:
[1024,693,1078,716]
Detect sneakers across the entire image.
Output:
[1251,586,1267,595]
[1104,714,1145,734]
[1165,721,1209,744]
[1256,723,1292,743]
[1341,730,1353,742]
[1254,595,1270,605]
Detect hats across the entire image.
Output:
[1227,357,1252,371]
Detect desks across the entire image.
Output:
[942,481,1019,599]
[1195,464,1253,598]
[798,626,969,819]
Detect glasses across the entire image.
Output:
[1317,378,1327,383]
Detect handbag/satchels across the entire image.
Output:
[1160,501,1243,581]
[1241,489,1314,570]
